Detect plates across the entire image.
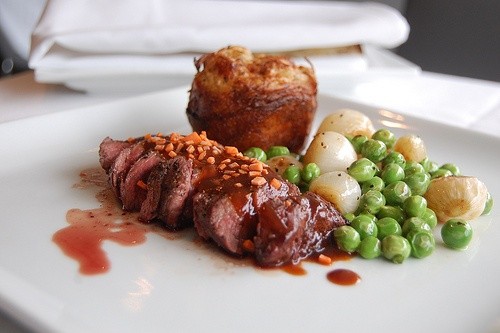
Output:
[1,86,500,332]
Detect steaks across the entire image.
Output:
[98,133,350,269]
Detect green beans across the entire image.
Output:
[243,129,493,263]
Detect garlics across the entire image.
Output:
[268,107,488,224]
